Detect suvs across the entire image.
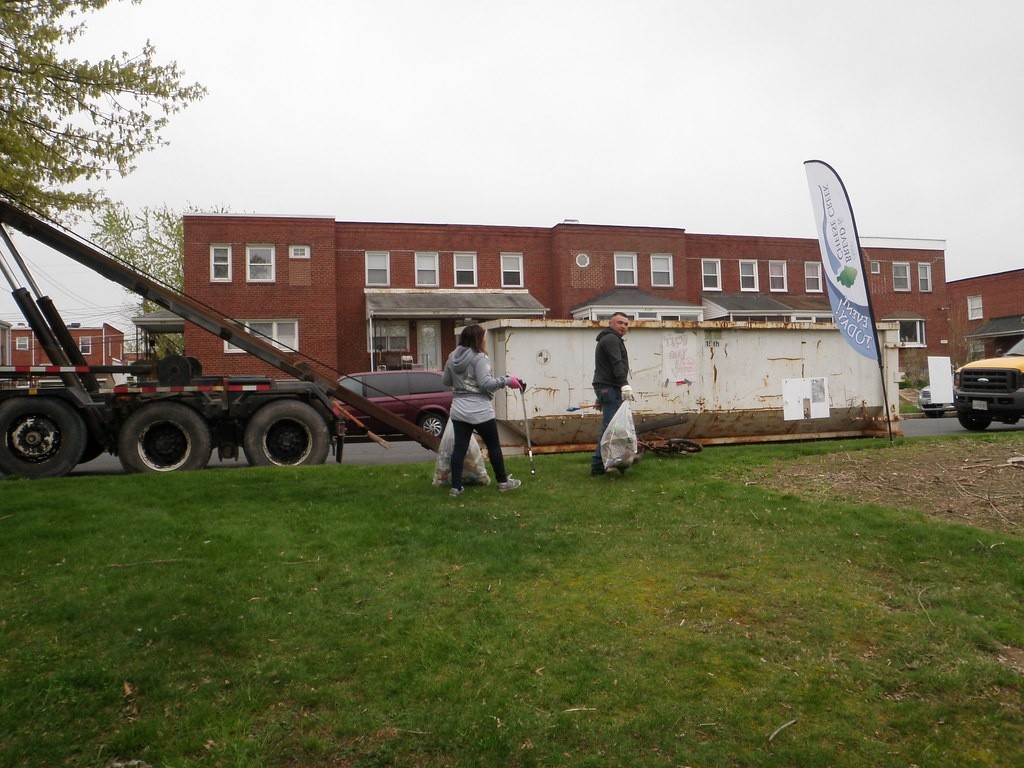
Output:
[953,338,1024,431]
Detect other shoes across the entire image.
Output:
[591,470,607,476]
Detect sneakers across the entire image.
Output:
[449,486,464,496]
[496,473,521,492]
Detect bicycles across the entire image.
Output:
[636,437,703,455]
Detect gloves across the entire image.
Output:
[593,400,604,413]
[507,376,522,390]
[621,385,635,402]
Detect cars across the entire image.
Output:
[916,386,954,419]
[327,370,453,439]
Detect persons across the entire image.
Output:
[442,324,524,498]
[589,311,636,477]
